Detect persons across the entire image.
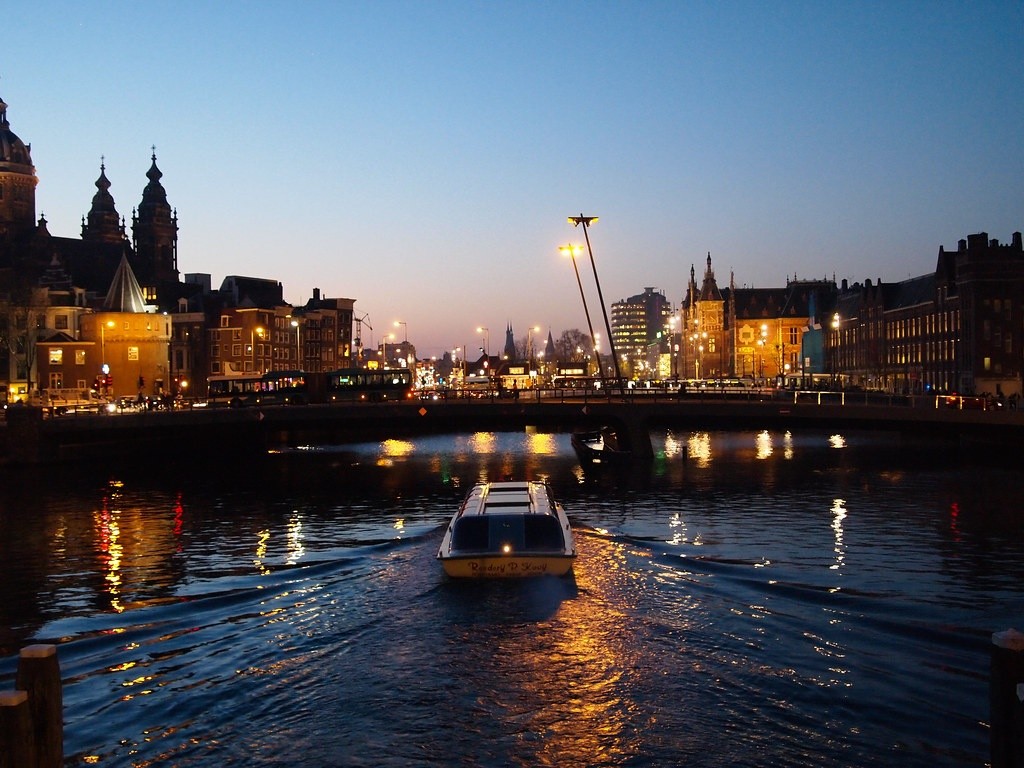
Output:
[496,379,503,399]
[685,378,762,390]
[978,389,1021,411]
[631,380,669,389]
[512,379,519,401]
[557,380,627,389]
[343,376,404,386]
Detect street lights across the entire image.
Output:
[102,364,110,395]
[399,321,409,369]
[482,327,490,390]
[291,320,301,371]
[251,326,263,376]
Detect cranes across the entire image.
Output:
[354,314,373,366]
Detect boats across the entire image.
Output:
[435,477,578,580]
[570,424,607,460]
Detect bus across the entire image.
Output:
[205,369,310,409]
[327,367,412,403]
